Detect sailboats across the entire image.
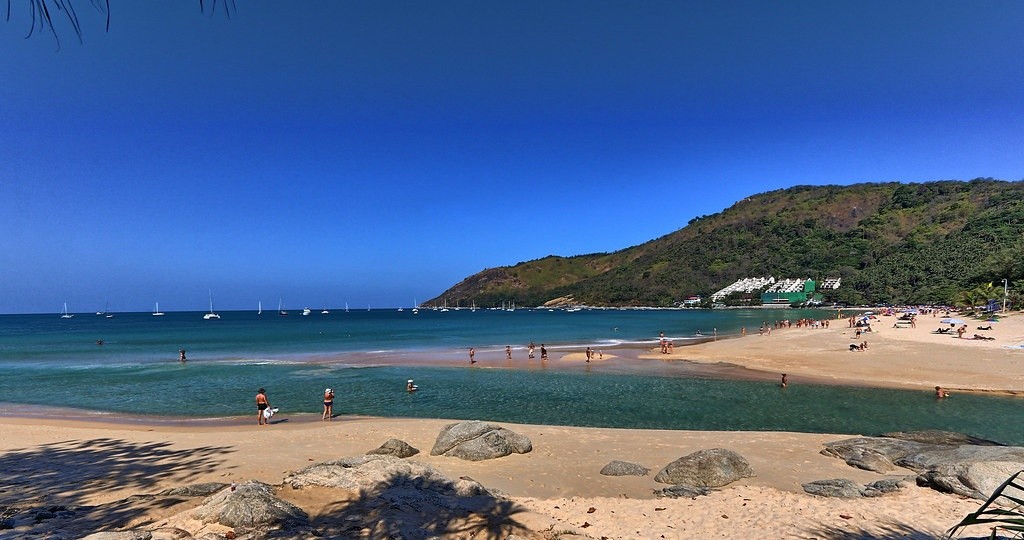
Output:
[397,296,516,315]
[277,295,289,315]
[367,305,370,312]
[345,301,350,313]
[257,300,263,315]
[59,301,75,319]
[202,288,221,320]
[152,301,165,316]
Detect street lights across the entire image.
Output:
[1001,278,1007,313]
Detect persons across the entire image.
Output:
[586,347,603,362]
[406,380,418,392]
[470,348,474,365]
[321,388,334,421]
[657,303,1000,355]
[505,340,549,361]
[256,388,270,425]
[782,373,787,391]
[935,386,944,398]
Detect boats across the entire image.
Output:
[548,307,581,313]
[940,318,964,323]
[95,311,117,319]
[299,306,330,316]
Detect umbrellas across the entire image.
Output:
[899,309,918,317]
[940,318,964,334]
